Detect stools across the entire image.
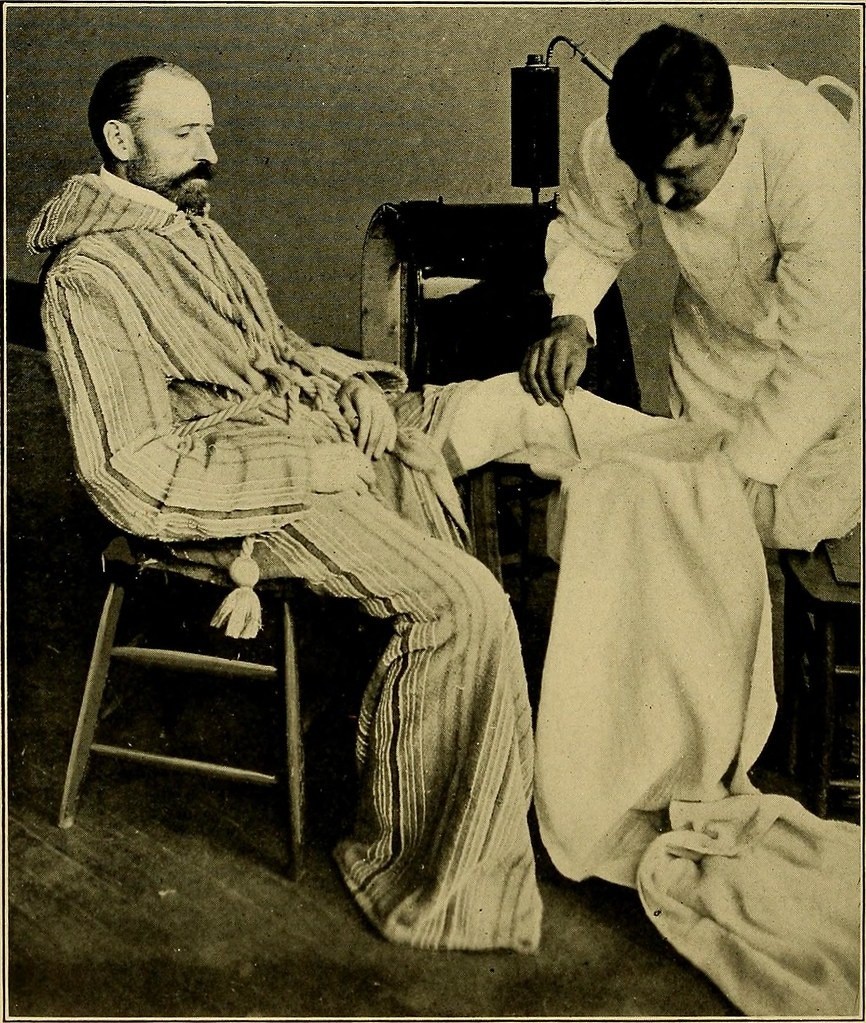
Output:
[58,536,308,881]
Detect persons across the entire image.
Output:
[520,24,861,823]
[25,57,727,952]
